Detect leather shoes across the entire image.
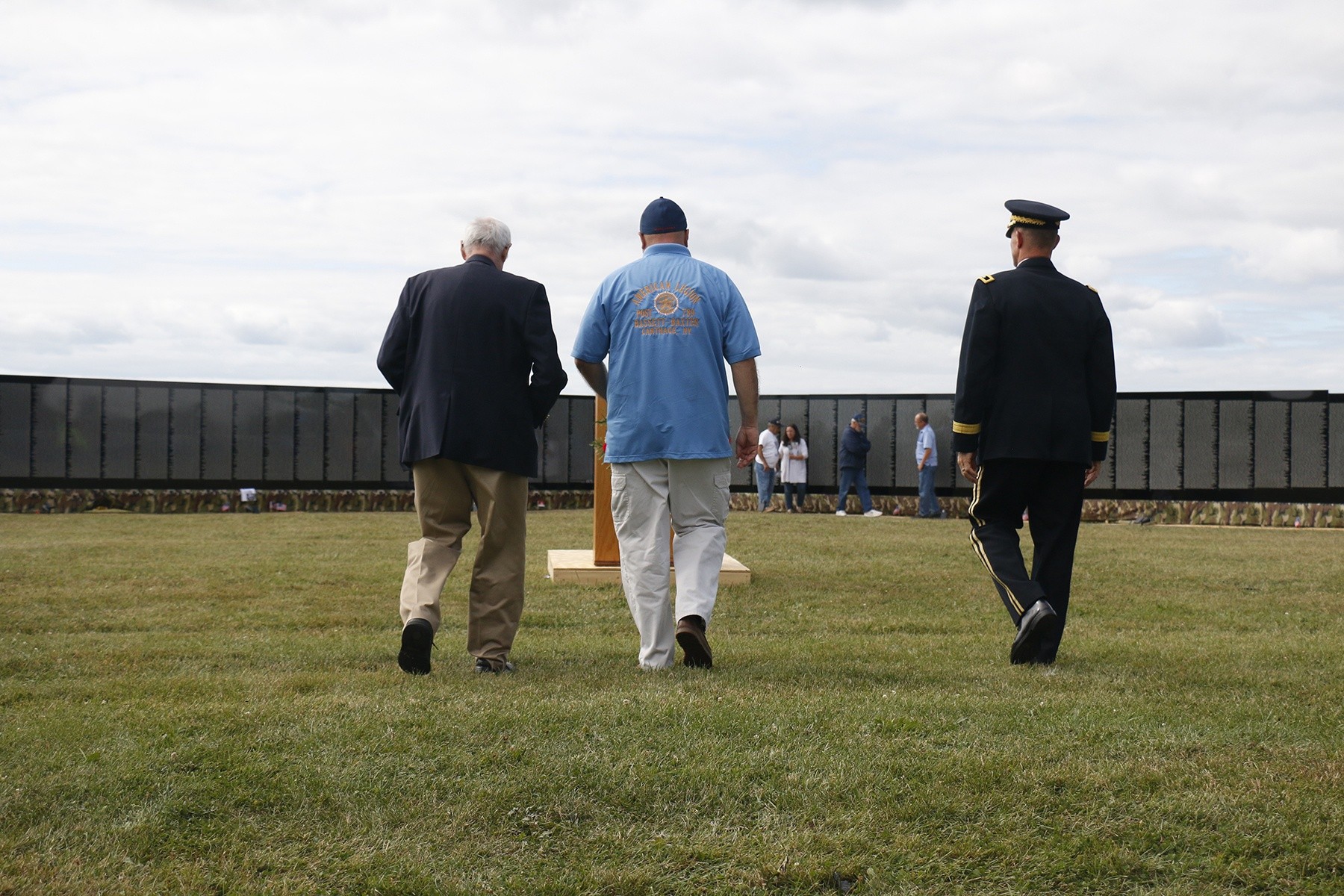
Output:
[1011,596,1058,664]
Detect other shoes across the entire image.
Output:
[398,619,439,675]
[787,508,793,514]
[763,506,775,513]
[914,510,942,518]
[864,509,883,517]
[675,617,713,670]
[796,506,802,512]
[475,658,514,677]
[835,510,847,516]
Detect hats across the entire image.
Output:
[768,416,782,426]
[639,196,687,234]
[853,413,866,427]
[1005,199,1070,238]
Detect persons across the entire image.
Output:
[950,201,1117,667]
[913,413,941,518]
[376,218,567,677]
[835,413,883,518]
[753,418,784,513]
[570,195,761,672]
[778,424,809,513]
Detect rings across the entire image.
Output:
[962,468,965,471]
